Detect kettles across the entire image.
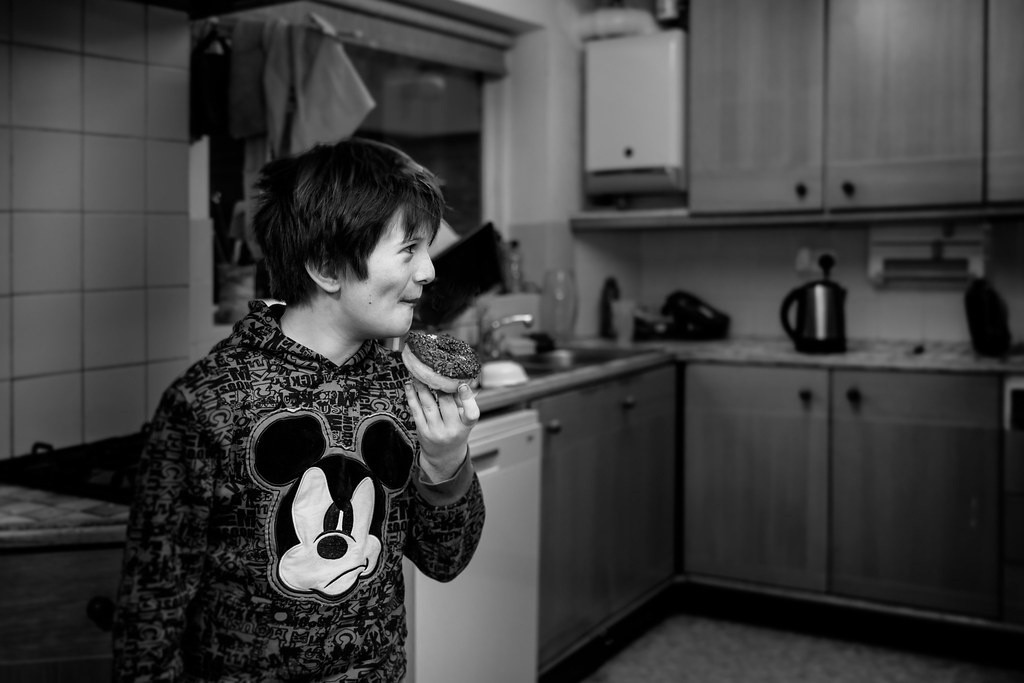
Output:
[779,280,851,356]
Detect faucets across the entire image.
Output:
[480,313,537,358]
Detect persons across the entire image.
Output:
[108,137,488,683]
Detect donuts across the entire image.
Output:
[402,331,481,393]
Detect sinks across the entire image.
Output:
[515,346,640,372]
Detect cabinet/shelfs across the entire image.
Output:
[684,362,1005,630]
[985,0,1024,215]
[529,358,678,683]
[686,0,985,216]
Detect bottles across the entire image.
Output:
[502,250,527,294]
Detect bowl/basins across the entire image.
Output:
[479,360,527,389]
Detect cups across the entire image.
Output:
[219,265,256,324]
[611,300,637,346]
